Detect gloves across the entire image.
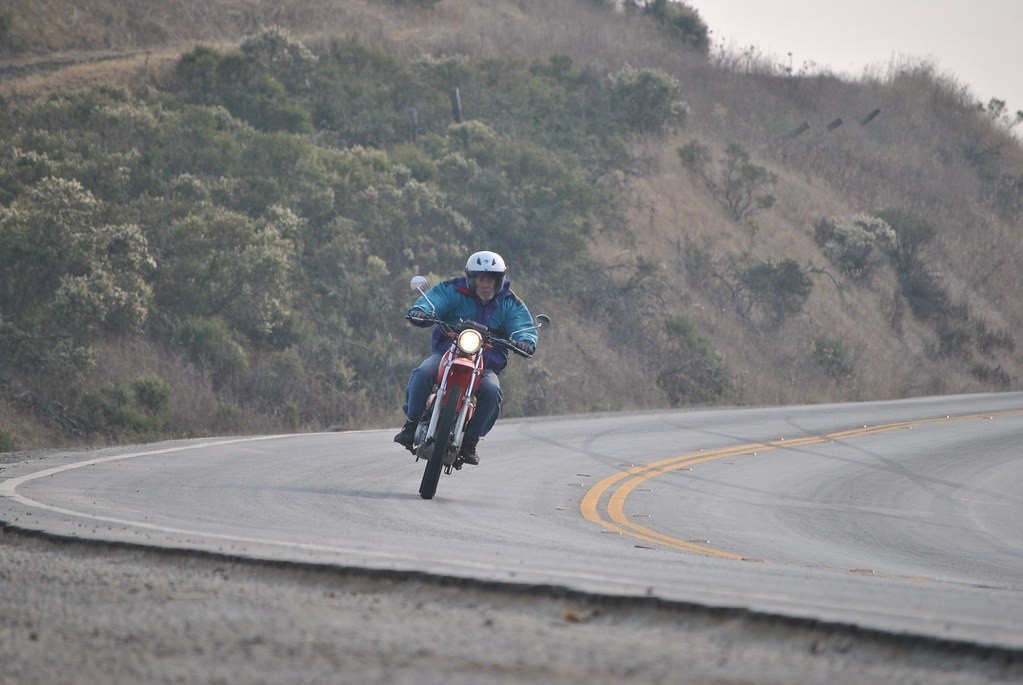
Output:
[409,310,425,318]
[517,341,534,353]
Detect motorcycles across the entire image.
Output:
[405,275,552,497]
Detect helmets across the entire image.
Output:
[465,250,507,295]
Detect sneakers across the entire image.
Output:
[458,431,481,465]
[394,420,418,443]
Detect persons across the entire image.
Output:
[393,250,538,465]
